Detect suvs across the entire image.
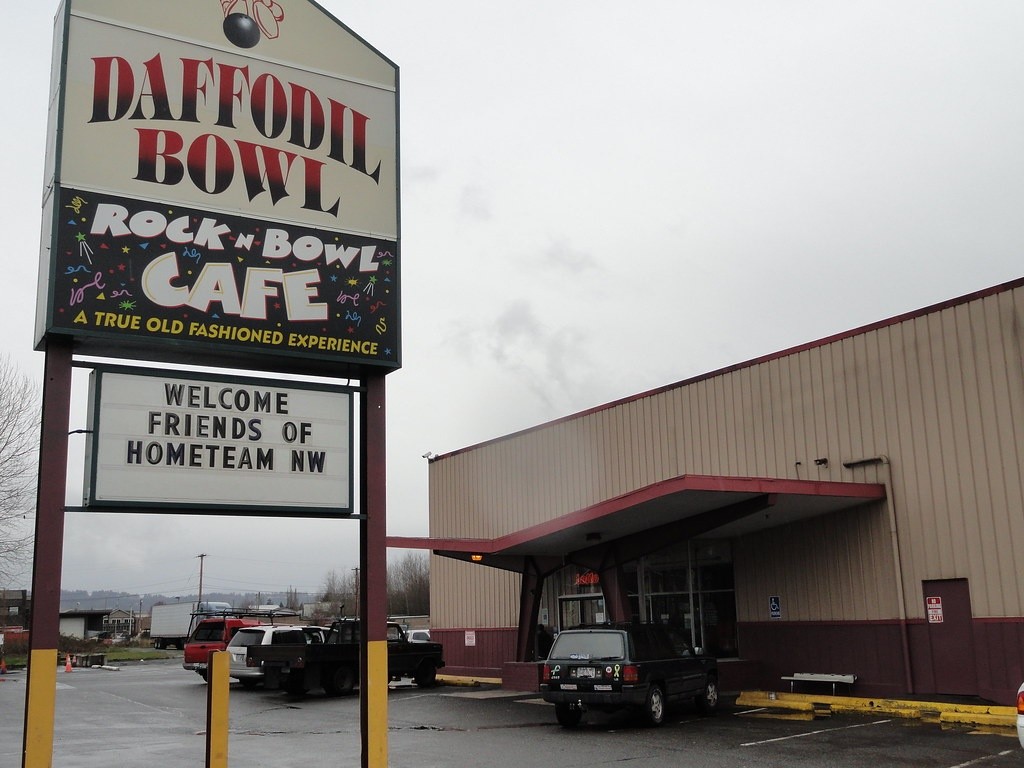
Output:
[184,617,261,681]
[538,620,721,727]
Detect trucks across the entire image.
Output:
[149,601,232,651]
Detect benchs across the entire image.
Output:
[781,672,858,696]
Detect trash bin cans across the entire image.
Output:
[68,654,106,667]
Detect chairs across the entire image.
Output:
[311,636,319,644]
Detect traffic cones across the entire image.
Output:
[0,660,7,675]
[63,654,74,673]
[72,653,78,663]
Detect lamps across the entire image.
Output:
[586,532,601,545]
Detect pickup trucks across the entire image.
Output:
[245,615,445,693]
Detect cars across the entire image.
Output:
[225,624,342,686]
[400,628,430,643]
